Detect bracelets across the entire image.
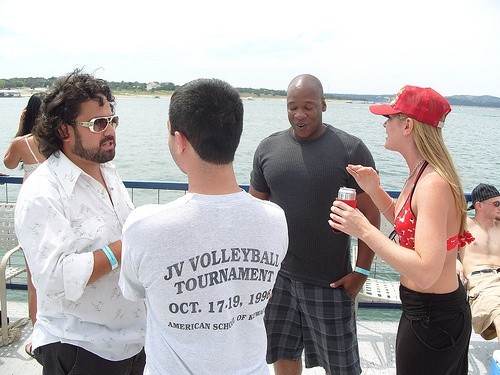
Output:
[101,245,118,271]
[381,198,394,214]
[354,266,370,276]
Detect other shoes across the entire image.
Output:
[25,343,35,358]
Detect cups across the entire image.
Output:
[330,187,357,235]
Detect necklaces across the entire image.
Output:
[407,158,426,181]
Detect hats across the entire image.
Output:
[467,183,500,211]
[369,85,452,129]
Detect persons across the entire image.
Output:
[12,65,145,373]
[117,76,290,375]
[4,91,50,325]
[247,73,380,375]
[327,85,472,375]
[454,182,500,348]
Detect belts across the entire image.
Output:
[471,268,500,275]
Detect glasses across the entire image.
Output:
[483,201,500,208]
[74,115,119,134]
